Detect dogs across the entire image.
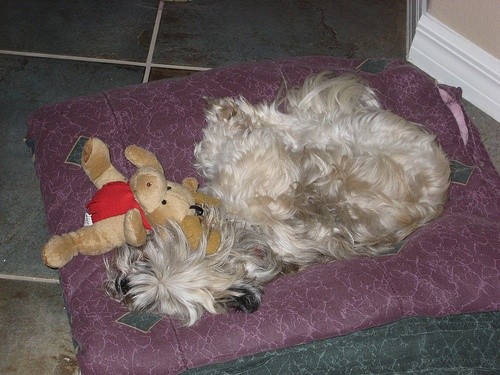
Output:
[103,69,452,330]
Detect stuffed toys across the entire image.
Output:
[42,135,224,270]
